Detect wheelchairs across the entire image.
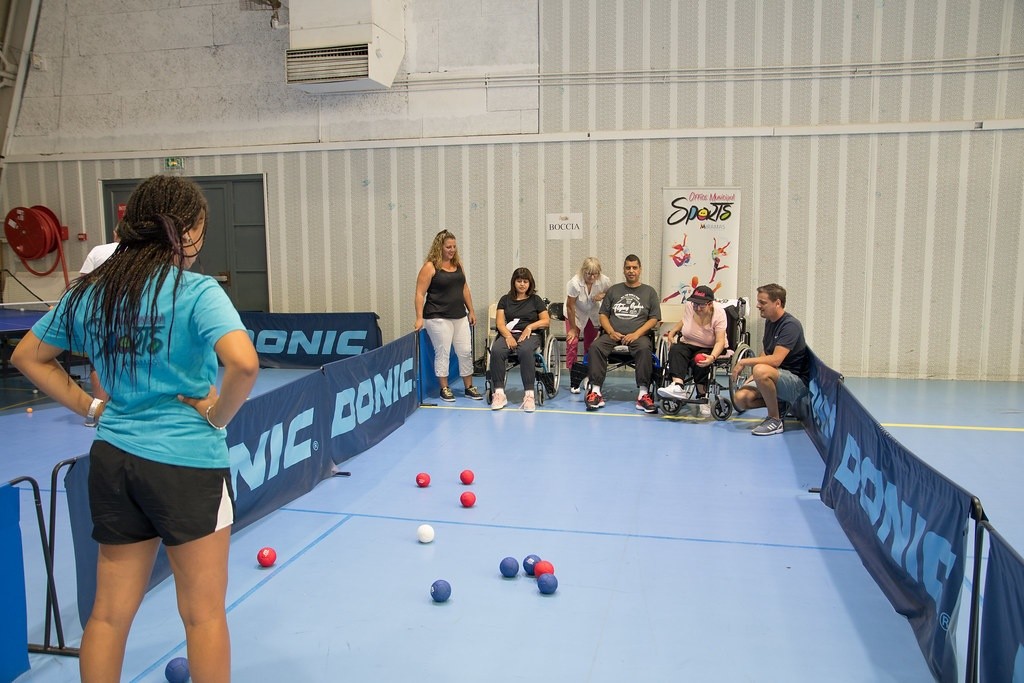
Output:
[658,297,755,422]
[483,299,562,407]
[584,320,672,415]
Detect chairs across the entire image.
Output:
[486,304,497,347]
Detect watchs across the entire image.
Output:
[84,398,104,428]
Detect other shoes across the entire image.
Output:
[84,421,97,427]
[570,387,580,394]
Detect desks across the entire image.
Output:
[549,320,684,340]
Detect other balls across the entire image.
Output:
[523,554,558,593]
[166,658,190,683]
[460,491,476,507]
[257,547,276,567]
[460,470,474,484]
[416,473,430,487]
[500,557,519,578]
[417,524,434,543]
[430,580,451,602]
[694,354,707,364]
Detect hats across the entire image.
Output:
[687,286,714,304]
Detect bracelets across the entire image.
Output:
[608,330,615,335]
[206,406,228,430]
[710,354,716,362]
[525,328,531,330]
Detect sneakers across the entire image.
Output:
[780,401,791,420]
[751,417,784,435]
[657,382,687,400]
[465,385,483,399]
[586,392,605,409]
[439,387,456,401]
[699,404,711,416]
[519,394,535,411]
[491,392,507,410]
[636,394,657,412]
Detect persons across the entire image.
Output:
[78,219,128,403]
[10,174,260,683]
[562,257,612,394]
[731,283,811,436]
[657,284,729,415]
[490,267,550,410]
[585,254,661,414]
[413,229,484,402]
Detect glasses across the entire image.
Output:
[691,301,710,308]
[584,272,600,277]
